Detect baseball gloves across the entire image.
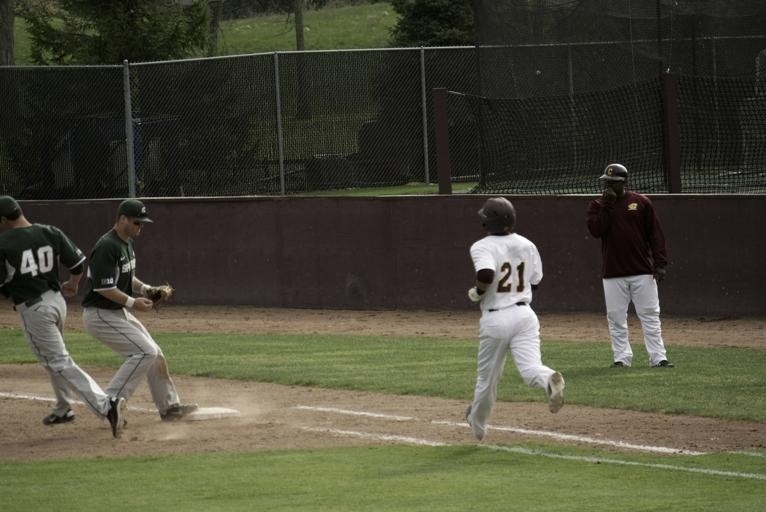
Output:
[146,286,173,307]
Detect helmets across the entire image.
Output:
[479,197,515,230]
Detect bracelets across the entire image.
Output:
[475,288,486,296]
[126,297,135,308]
[140,284,150,296]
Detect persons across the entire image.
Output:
[464,197,565,441]
[0,196,126,438]
[81,198,198,423]
[586,163,674,368]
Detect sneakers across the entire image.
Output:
[106,397,126,438]
[160,402,195,422]
[43,409,75,423]
[652,359,674,369]
[548,371,563,412]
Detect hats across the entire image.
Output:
[118,198,152,223]
[1,194,18,216]
[600,163,630,181]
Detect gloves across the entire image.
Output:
[654,261,667,284]
[469,285,481,304]
[601,186,616,203]
